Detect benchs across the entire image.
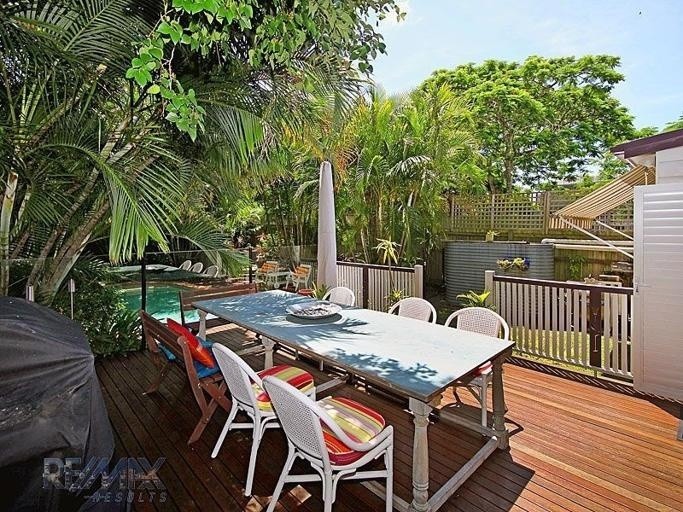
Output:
[178,282,261,338]
[140,310,247,445]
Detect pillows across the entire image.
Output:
[167,317,214,368]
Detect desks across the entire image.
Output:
[191,290,516,512]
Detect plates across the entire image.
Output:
[286,301,342,321]
[141,265,168,272]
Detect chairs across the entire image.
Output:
[261,375,394,512]
[211,342,316,496]
[178,260,219,277]
[388,297,437,325]
[322,287,355,306]
[444,306,508,438]
[254,261,312,292]
